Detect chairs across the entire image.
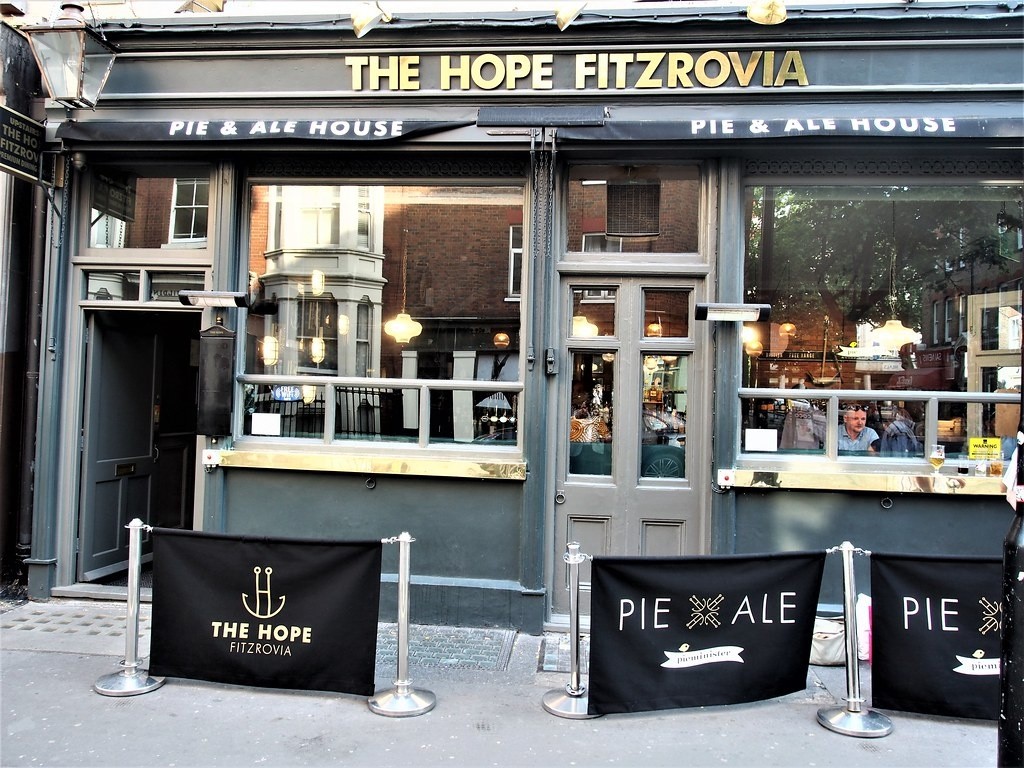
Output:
[780,411,821,450]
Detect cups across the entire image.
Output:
[989,450,1004,476]
[958,455,968,475]
[975,449,987,477]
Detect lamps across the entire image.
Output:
[780,266,797,336]
[383,231,422,343]
[494,333,510,349]
[647,301,663,336]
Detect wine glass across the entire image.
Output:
[929,445,946,475]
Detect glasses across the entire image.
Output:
[847,405,870,411]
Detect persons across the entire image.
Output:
[780,384,826,449]
[880,385,925,458]
[838,404,880,450]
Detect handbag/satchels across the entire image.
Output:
[809,618,846,664]
[778,399,819,450]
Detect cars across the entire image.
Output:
[471,408,684,478]
